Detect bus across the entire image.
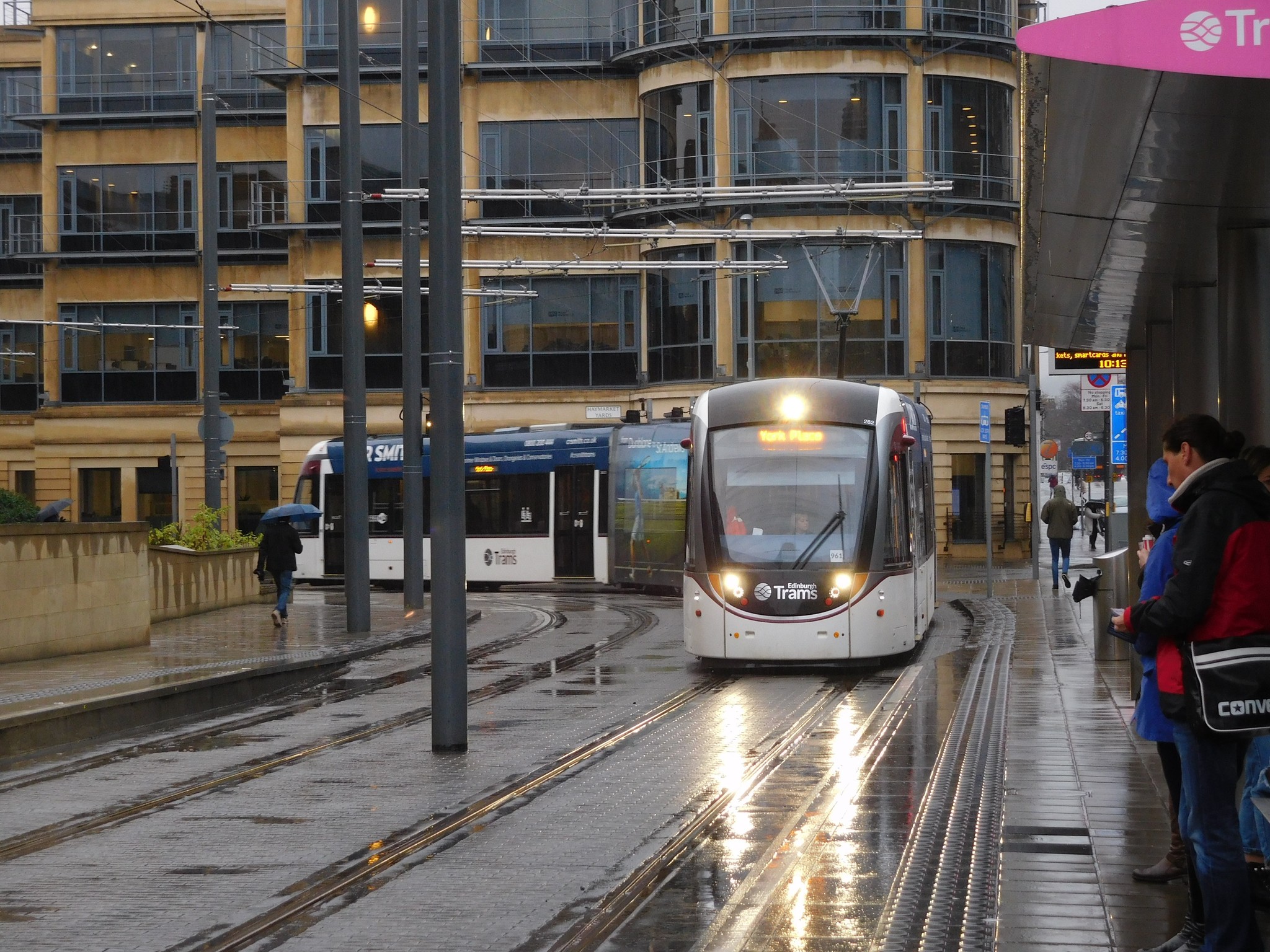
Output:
[286,239,939,668]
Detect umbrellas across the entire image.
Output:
[36,499,74,523]
[261,503,323,523]
[1084,501,1106,508]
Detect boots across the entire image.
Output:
[1139,884,1204,952]
[1132,797,1187,882]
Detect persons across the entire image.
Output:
[1085,504,1103,549]
[1048,473,1058,499]
[1041,485,1078,589]
[1077,478,1088,505]
[791,514,813,535]
[1111,414,1270,952]
[43,513,65,522]
[1074,469,1080,487]
[1238,450,1270,919]
[1130,456,1184,878]
[256,516,303,627]
[628,469,653,579]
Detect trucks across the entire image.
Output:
[1072,436,1125,482]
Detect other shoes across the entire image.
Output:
[1062,573,1071,588]
[1053,585,1058,590]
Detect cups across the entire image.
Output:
[1143,535,1156,552]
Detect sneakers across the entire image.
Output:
[281,618,289,624]
[271,610,282,627]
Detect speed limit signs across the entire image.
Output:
[1085,431,1094,440]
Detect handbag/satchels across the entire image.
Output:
[1184,630,1270,737]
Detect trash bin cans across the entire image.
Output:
[1091,546,1129,661]
